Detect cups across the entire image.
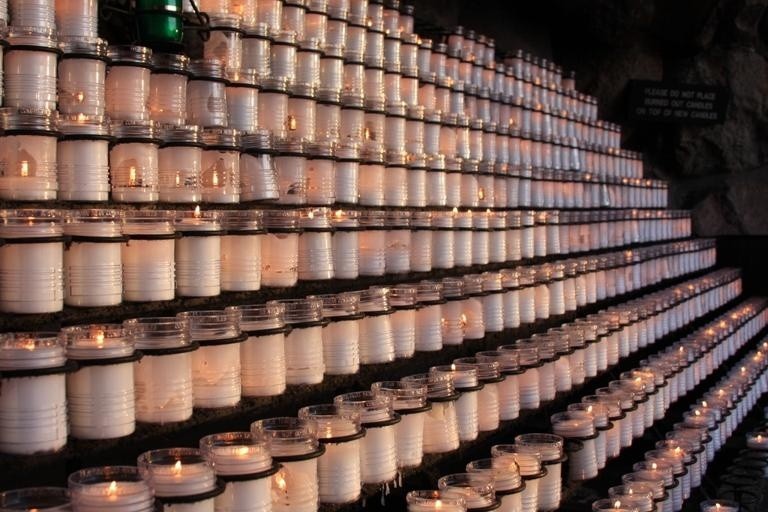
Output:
[0,1,767,511]
